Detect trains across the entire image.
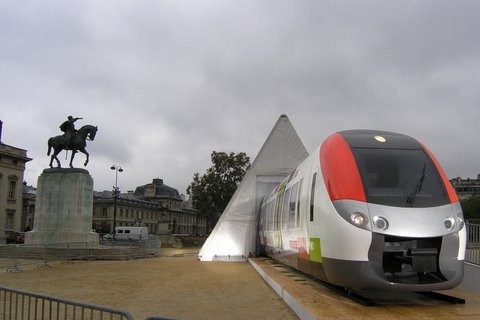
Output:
[258,130,467,291]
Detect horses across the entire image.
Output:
[47,124,98,168]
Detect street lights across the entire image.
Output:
[111,164,123,239]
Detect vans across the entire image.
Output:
[6,232,25,244]
[104,226,148,241]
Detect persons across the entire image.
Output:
[24,226,31,231]
[172,216,177,234]
[60,116,78,153]
